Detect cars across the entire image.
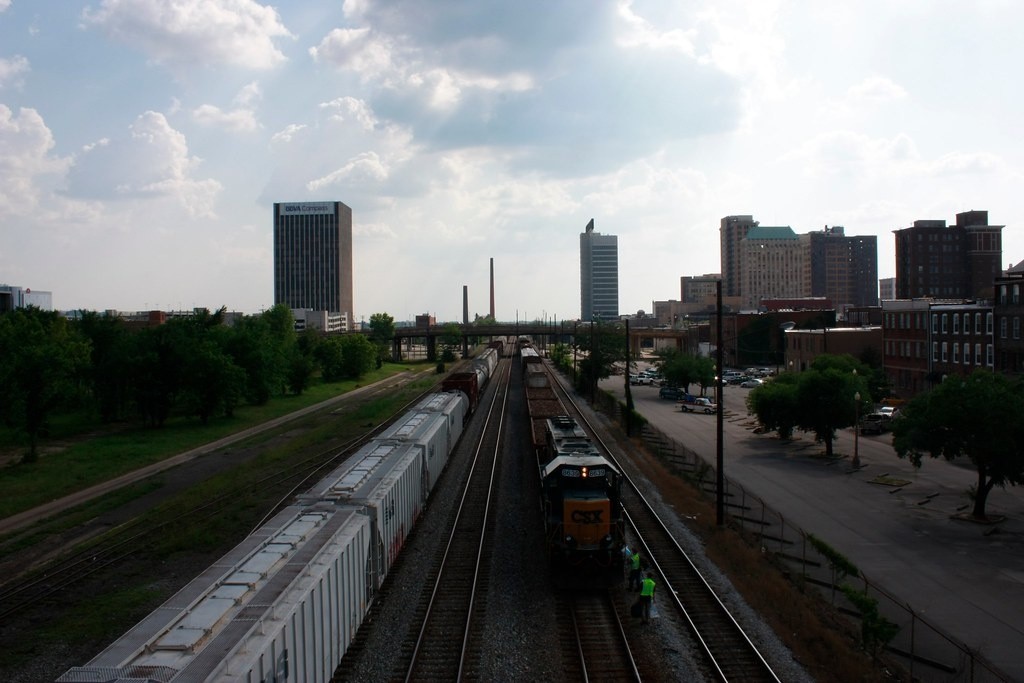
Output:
[710,361,779,391]
[878,405,898,418]
[629,367,662,386]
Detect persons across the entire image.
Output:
[634,572,658,626]
[626,547,645,592]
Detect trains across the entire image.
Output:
[46,328,512,683]
[517,331,623,599]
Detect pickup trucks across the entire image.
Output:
[681,396,717,414]
